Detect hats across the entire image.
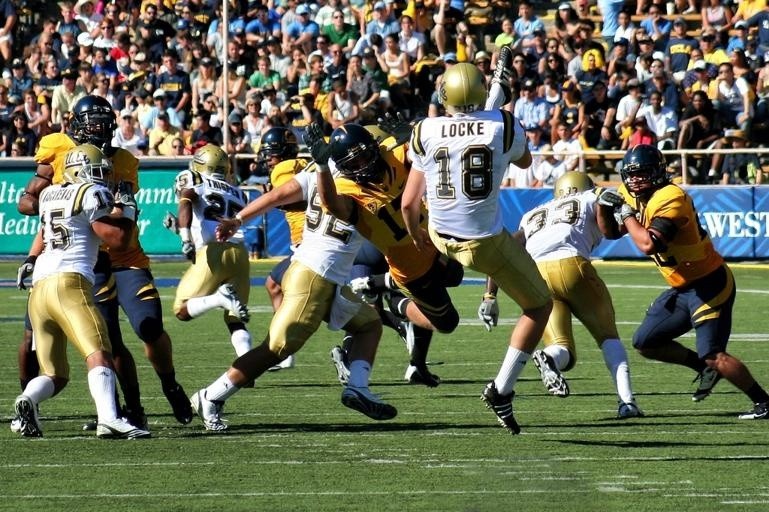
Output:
[11,20,212,119]
[295,4,311,16]
[734,20,749,30]
[673,17,686,27]
[374,2,385,11]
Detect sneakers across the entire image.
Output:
[737,399,769,421]
[9,378,229,439]
[335,385,400,423]
[689,364,724,403]
[531,348,570,400]
[328,322,444,390]
[616,396,649,418]
[478,379,523,437]
[212,281,251,326]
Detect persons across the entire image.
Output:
[399,61,554,437]
[476,168,644,419]
[594,141,769,421]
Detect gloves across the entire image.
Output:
[595,186,624,208]
[14,255,39,291]
[162,210,180,235]
[376,110,415,156]
[300,121,338,166]
[181,240,198,265]
[476,293,500,331]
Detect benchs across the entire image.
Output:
[2,1,768,184]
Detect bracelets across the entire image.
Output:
[483,293,496,299]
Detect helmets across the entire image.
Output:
[619,142,668,199]
[437,61,489,114]
[256,127,298,165]
[67,94,118,146]
[64,143,113,188]
[553,169,595,199]
[188,143,229,180]
[328,122,386,183]
